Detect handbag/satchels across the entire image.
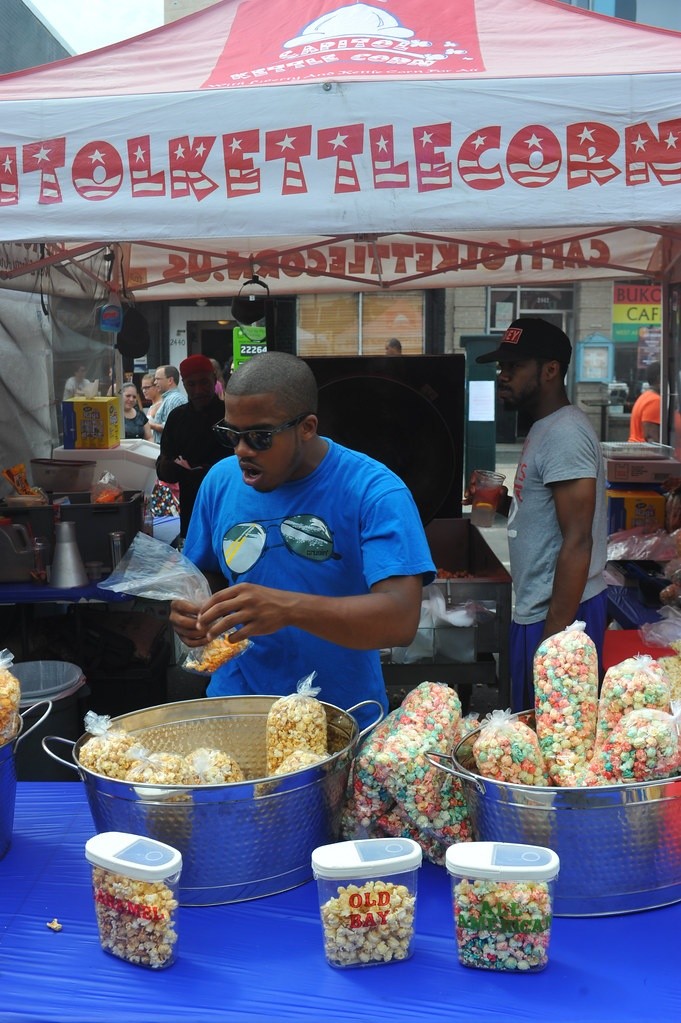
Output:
[114,307,149,358]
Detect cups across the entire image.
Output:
[471,470,506,527]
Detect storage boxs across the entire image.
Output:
[0,487,145,583]
[600,440,681,537]
[63,396,121,449]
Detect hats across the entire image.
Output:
[476,318,572,364]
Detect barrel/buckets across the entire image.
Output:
[423,704,681,917]
[0,700,56,859]
[42,693,383,906]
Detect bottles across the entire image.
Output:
[309,836,423,970]
[446,840,561,972]
[31,537,51,586]
[84,828,185,970]
[108,531,127,573]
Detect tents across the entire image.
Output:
[0,0,681,439]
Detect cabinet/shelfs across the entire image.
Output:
[379,519,514,708]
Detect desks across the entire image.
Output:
[581,398,616,443]
[0,575,134,667]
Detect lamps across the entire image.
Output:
[196,298,209,306]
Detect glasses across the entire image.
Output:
[141,384,156,390]
[212,412,318,451]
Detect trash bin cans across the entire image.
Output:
[8,660,86,782]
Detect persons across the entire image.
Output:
[169,351,437,729]
[462,317,608,713]
[628,362,660,442]
[62,358,235,444]
[385,338,402,354]
[155,354,235,539]
